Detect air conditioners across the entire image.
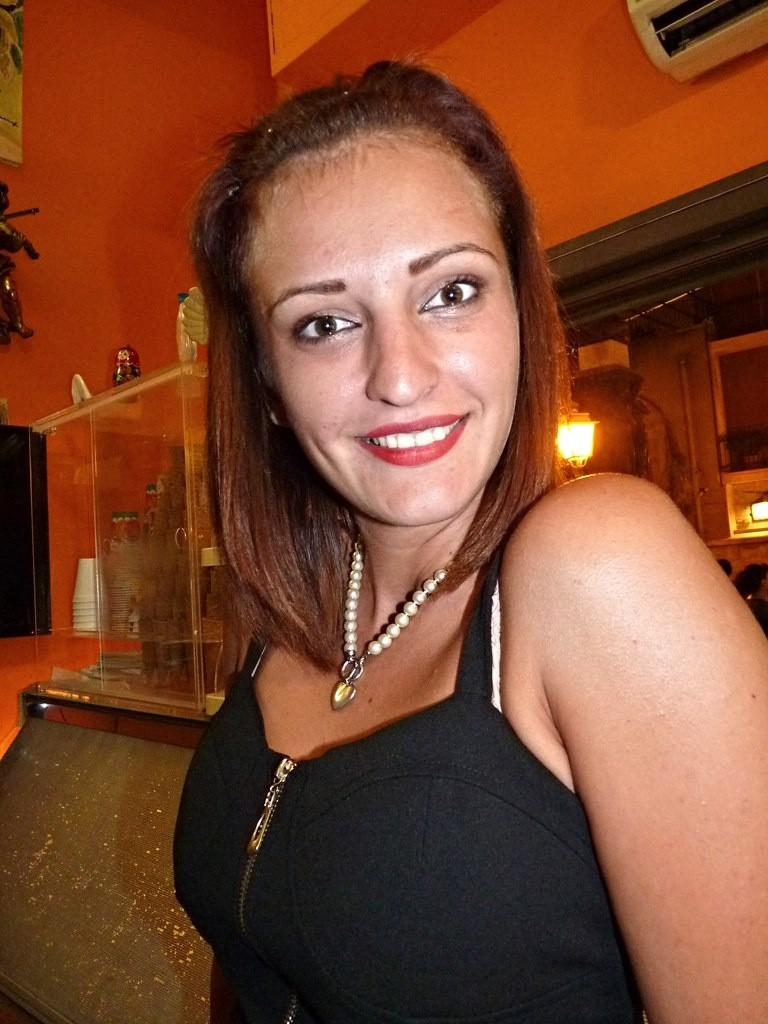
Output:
[626,0,768,84]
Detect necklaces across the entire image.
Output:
[332,530,456,713]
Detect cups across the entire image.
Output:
[72,484,158,634]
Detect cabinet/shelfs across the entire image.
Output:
[547,159,768,639]
[28,363,243,720]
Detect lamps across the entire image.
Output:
[555,410,599,468]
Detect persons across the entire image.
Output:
[170,58,768,1024]
